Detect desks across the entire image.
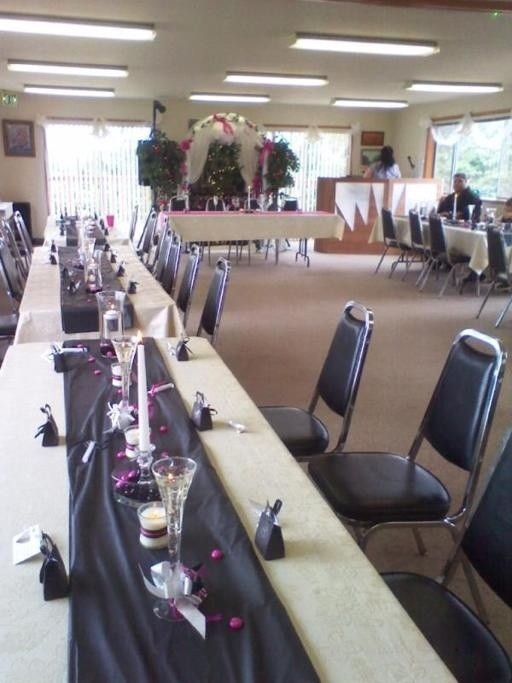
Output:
[161,209,344,268]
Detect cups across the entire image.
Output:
[82,249,104,293]
[137,501,170,549]
[124,425,151,458]
[477,224,486,231]
[96,291,126,346]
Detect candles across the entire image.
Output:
[248,186,250,209]
[139,507,168,548]
[113,366,132,386]
[138,329,150,449]
[103,303,122,339]
[452,193,456,220]
[154,467,194,573]
[118,346,132,409]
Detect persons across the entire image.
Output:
[364,145,402,179]
[437,172,481,222]
[499,199,512,222]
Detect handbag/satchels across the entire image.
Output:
[38,533,69,600]
[104,244,109,251]
[95,214,97,220]
[102,222,104,230]
[61,214,63,219]
[256,502,286,562]
[64,267,68,280]
[128,280,139,294]
[118,265,125,277]
[60,228,64,236]
[176,337,193,361]
[51,255,56,264]
[100,219,103,225]
[52,343,68,373]
[35,404,60,447]
[111,253,117,264]
[52,244,56,252]
[192,391,213,432]
[105,228,108,235]
[69,279,77,296]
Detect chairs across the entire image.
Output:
[257,300,374,462]
[196,257,232,347]
[1,209,34,340]
[400,209,442,285]
[129,202,183,296]
[415,215,470,299]
[475,224,511,327]
[380,427,511,682]
[307,328,508,625]
[373,206,425,278]
[176,242,203,328]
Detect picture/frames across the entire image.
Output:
[2,118,36,157]
[361,131,383,145]
[360,148,382,165]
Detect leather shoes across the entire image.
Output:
[464,272,486,282]
[424,259,441,270]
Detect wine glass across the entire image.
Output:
[257,195,265,213]
[111,335,140,412]
[486,208,496,217]
[84,238,96,264]
[152,457,196,622]
[232,198,239,211]
[213,196,218,212]
